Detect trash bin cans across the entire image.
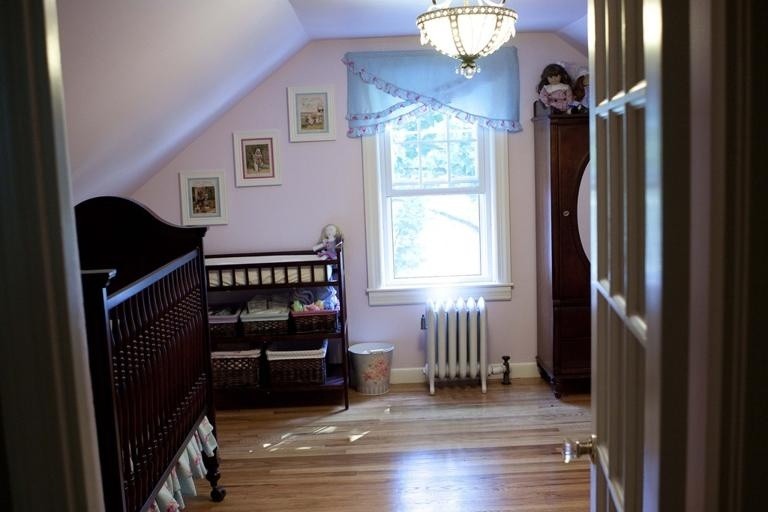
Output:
[346,342,396,397]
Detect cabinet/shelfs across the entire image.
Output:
[532,102,591,398]
[204,240,351,409]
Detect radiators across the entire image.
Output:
[417,296,511,396]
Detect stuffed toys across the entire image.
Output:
[317,223,345,261]
[538,64,585,116]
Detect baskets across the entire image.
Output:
[240,304,289,336]
[206,303,242,338]
[291,306,339,334]
[207,341,263,390]
[265,337,330,385]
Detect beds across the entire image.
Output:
[71,194,230,511]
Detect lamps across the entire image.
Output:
[414,0,514,75]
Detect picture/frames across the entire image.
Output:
[232,128,281,189]
[176,168,227,228]
[287,83,335,145]
